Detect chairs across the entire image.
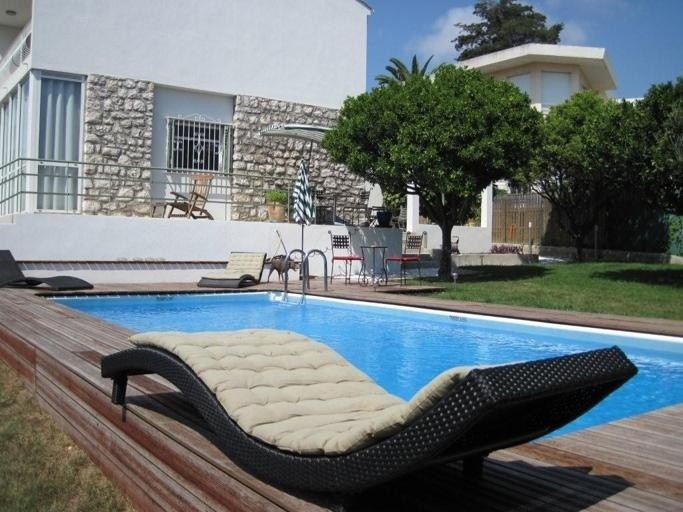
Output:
[315,187,373,226]
[154,173,214,220]
[0,246,93,292]
[198,251,268,288]
[328,230,429,286]
[98,327,639,510]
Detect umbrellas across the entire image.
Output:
[294,158,315,277]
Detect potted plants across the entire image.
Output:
[266,190,288,223]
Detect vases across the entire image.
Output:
[376,211,393,225]
